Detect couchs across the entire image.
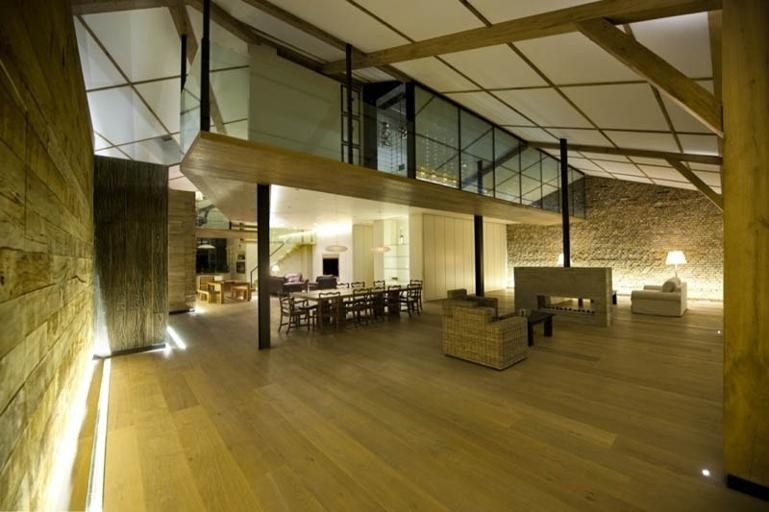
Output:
[441,288,530,370]
[269,271,337,297]
[630,276,688,318]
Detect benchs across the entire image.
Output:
[196,275,251,304]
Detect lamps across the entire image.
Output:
[665,250,687,279]
[196,238,215,251]
[271,260,281,272]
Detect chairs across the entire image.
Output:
[276,279,424,336]
